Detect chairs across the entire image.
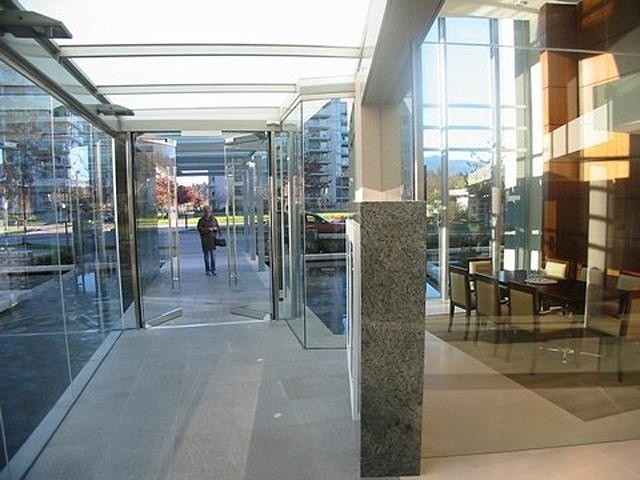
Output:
[443,254,639,381]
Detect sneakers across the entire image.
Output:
[205,270,217,277]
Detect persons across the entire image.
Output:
[197,206,226,276]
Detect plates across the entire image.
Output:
[525,278,558,285]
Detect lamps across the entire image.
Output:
[96,99,136,123]
[0,7,73,48]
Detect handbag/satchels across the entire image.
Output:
[214,224,227,247]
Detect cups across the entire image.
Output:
[527,267,548,282]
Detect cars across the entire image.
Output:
[275,207,347,234]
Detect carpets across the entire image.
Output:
[423,312,639,421]
